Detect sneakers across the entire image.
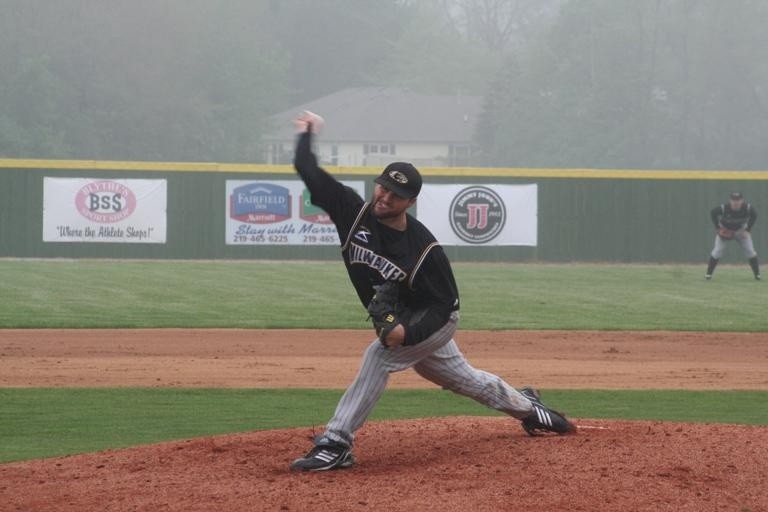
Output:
[518,385,576,437]
[287,434,356,475]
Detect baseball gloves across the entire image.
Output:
[368,283,411,346]
[718,228,733,240]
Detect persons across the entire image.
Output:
[702,189,763,283]
[287,106,578,476]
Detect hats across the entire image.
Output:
[729,190,744,202]
[374,161,423,199]
